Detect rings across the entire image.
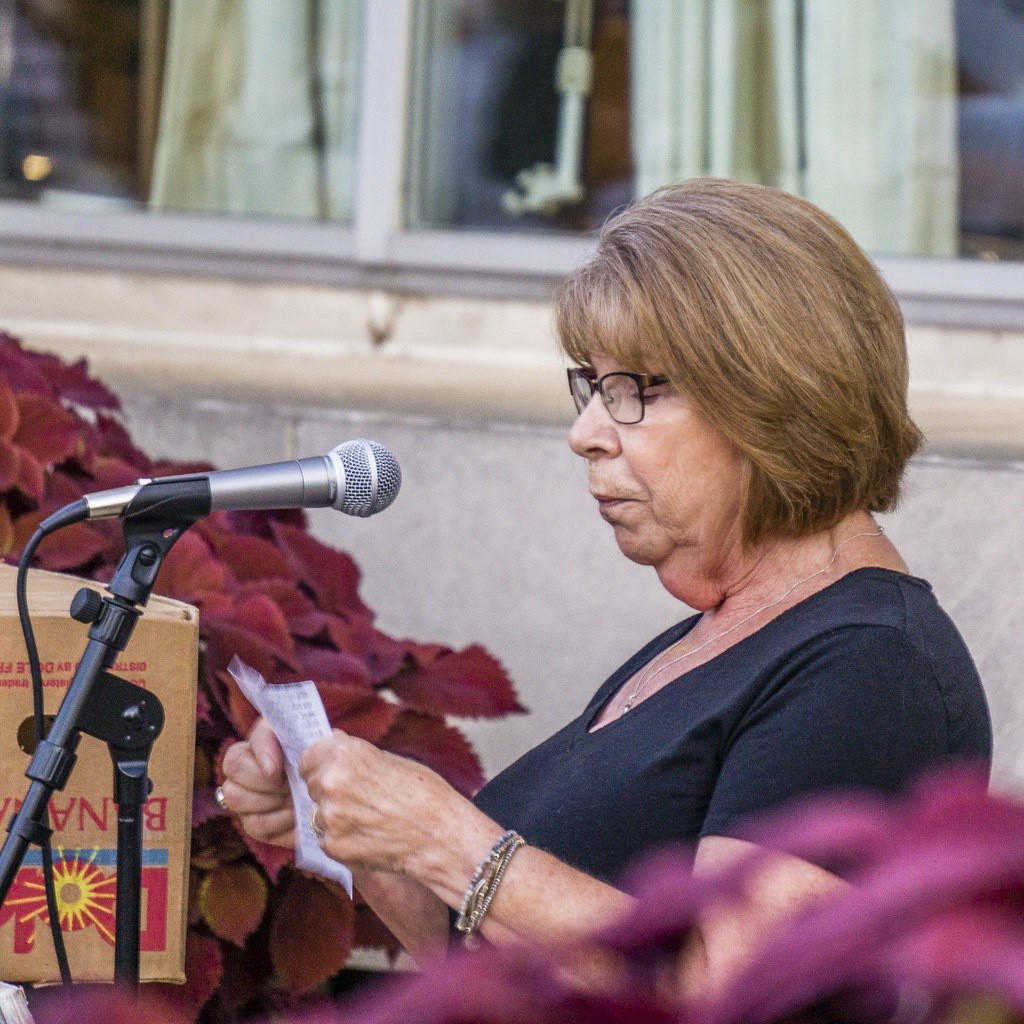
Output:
[311,810,324,838]
[215,787,228,809]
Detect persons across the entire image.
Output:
[222,177,994,1024]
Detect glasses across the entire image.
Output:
[567,367,685,424]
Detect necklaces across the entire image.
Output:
[621,526,886,717]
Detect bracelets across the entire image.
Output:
[457,830,526,951]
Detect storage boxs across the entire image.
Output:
[0,559,201,991]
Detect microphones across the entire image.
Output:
[84,438,400,520]
